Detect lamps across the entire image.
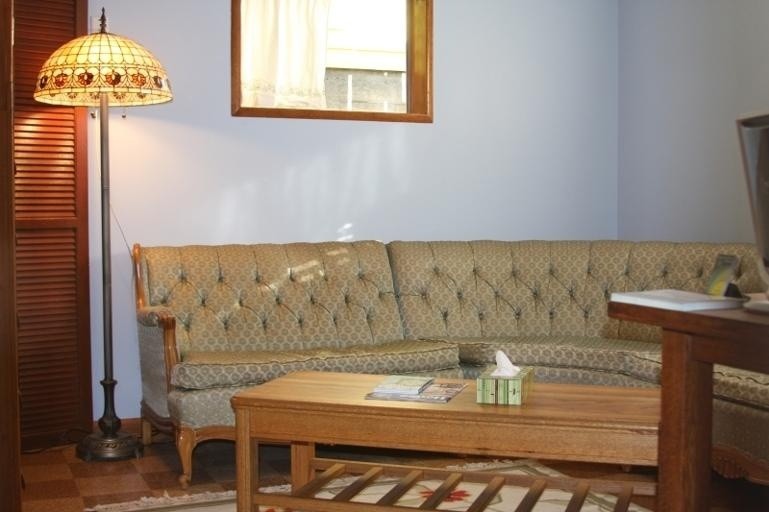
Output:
[32,2,172,459]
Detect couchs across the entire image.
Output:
[132,239,769,489]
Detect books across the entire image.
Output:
[365,383,469,403]
[610,288,749,313]
[374,375,435,394]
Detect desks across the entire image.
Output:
[607,291,769,511]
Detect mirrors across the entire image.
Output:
[230,0,434,123]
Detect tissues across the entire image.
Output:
[475,348,535,406]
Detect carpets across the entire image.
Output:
[86,455,653,512]
